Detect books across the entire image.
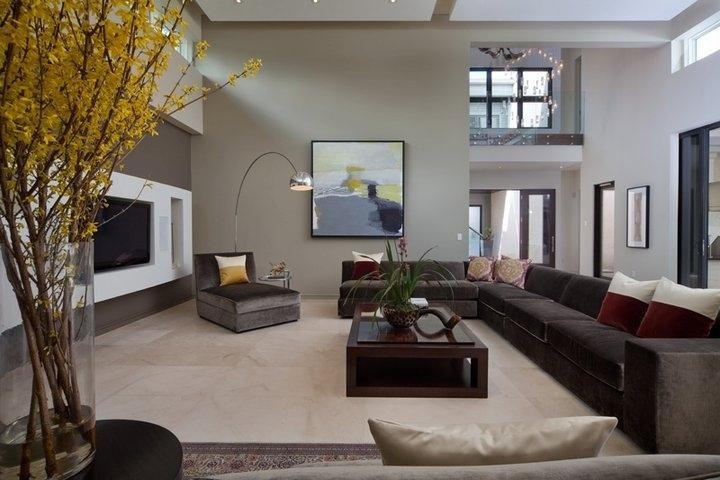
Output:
[409,297,429,311]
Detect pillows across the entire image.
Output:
[594,271,658,334]
[349,251,384,280]
[366,416,621,467]
[495,255,532,290]
[634,278,719,340]
[466,256,496,282]
[215,255,251,287]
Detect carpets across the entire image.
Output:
[177,442,383,479]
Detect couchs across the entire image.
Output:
[337,260,719,455]
[194,252,299,334]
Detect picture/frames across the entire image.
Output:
[309,140,406,237]
[627,185,650,249]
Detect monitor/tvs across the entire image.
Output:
[91,199,151,271]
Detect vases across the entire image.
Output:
[385,329,417,342]
[381,303,422,328]
[0,244,95,480]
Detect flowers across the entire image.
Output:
[342,230,461,309]
[0,0,260,480]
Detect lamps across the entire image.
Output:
[234,151,314,253]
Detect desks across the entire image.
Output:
[91,418,183,480]
[258,273,292,290]
